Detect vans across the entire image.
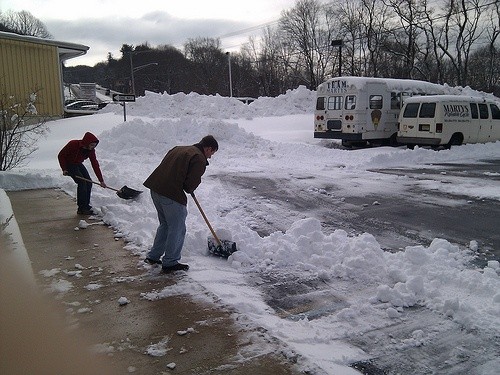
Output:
[396,95,500,149]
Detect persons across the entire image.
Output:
[144,135,218,272]
[58,132,106,215]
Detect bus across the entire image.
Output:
[313,75,460,149]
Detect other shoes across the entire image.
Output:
[144,258,162,265]
[160,263,189,274]
[78,206,92,211]
[77,210,94,215]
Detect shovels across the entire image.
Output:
[66,173,144,200]
[191,193,236,258]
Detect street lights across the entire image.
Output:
[330,40,344,76]
[131,63,158,94]
